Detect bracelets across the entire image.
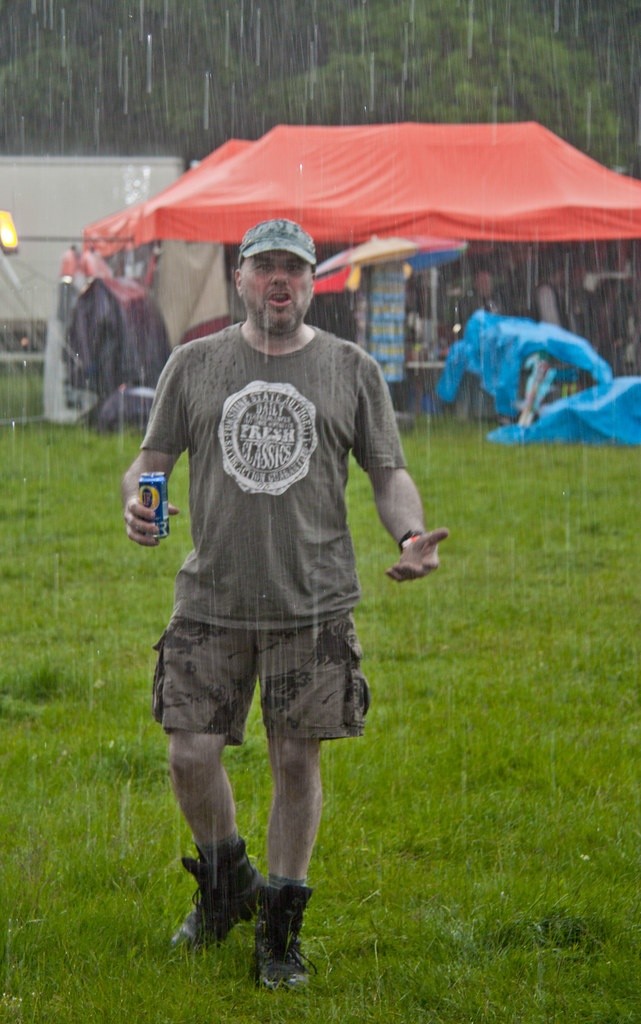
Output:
[398,530,423,551]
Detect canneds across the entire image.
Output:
[138,471,169,538]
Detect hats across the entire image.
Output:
[238,218,316,274]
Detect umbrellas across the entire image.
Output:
[310,232,465,296]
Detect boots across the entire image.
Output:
[249,883,317,997]
[172,836,267,954]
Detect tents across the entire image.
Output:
[83,120,641,349]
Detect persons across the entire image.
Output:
[120,217,450,989]
